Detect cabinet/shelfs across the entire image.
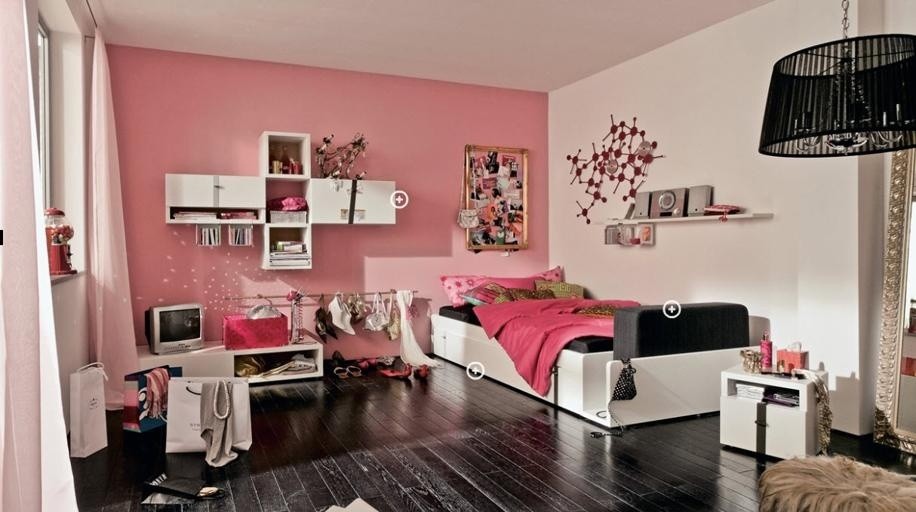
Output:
[165,132,396,269]
[107,331,324,384]
[719,362,828,460]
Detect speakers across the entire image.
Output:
[687,185,712,216]
[634,192,651,219]
[650,188,687,218]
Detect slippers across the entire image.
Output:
[332,365,363,380]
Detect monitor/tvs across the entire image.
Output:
[144,303,206,356]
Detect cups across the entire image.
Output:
[291,159,300,174]
[270,159,283,175]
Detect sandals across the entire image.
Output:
[414,364,429,380]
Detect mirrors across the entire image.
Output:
[873,150,916,454]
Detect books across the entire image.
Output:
[230,227,251,245]
[172,210,256,219]
[734,382,798,407]
[270,252,309,266]
[198,227,218,244]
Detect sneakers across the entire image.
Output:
[354,356,379,369]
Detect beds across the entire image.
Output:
[431,303,750,430]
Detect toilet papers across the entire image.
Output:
[776,342,808,369]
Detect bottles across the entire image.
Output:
[759,329,773,369]
[907,298,916,335]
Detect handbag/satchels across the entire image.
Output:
[68,361,109,460]
[121,364,171,434]
[163,376,254,455]
[364,291,388,334]
[386,289,401,343]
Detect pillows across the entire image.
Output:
[440,267,584,309]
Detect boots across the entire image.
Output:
[312,290,366,345]
[380,363,413,379]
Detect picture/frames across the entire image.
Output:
[465,144,528,250]
[639,223,653,246]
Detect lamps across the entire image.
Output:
[758,0,916,158]
